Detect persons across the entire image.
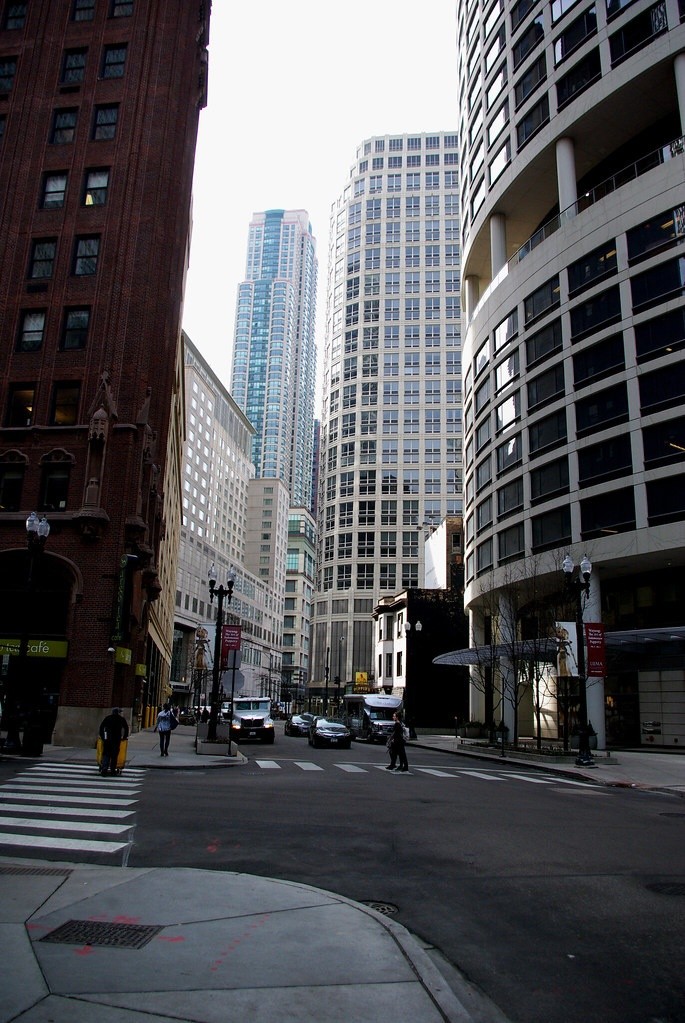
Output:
[201,707,209,723]
[154,703,174,757]
[99,707,129,776]
[385,711,409,772]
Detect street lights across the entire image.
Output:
[405,620,422,742]
[563,552,598,770]
[202,562,237,743]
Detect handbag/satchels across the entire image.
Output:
[169,711,179,731]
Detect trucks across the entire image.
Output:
[343,693,404,744]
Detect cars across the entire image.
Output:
[194,701,233,719]
[308,716,351,751]
[283,713,314,737]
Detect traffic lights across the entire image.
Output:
[334,675,339,685]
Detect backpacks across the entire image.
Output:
[398,724,409,740]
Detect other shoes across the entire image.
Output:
[161,753,163,756]
[110,770,117,776]
[401,766,409,771]
[386,763,397,769]
[396,766,403,770]
[164,751,168,755]
[102,768,107,778]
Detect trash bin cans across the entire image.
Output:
[96,737,128,769]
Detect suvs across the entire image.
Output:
[227,696,276,745]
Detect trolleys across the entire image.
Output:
[96,736,128,774]
